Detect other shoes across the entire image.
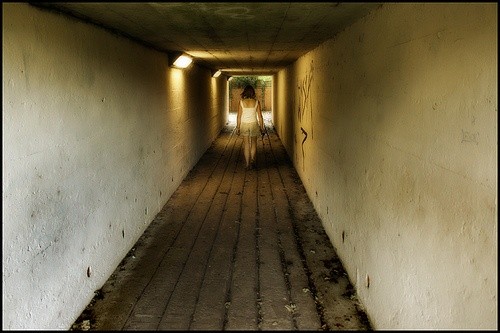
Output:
[246,161,255,170]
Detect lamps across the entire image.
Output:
[167,52,194,71]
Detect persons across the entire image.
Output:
[236,83,266,170]
